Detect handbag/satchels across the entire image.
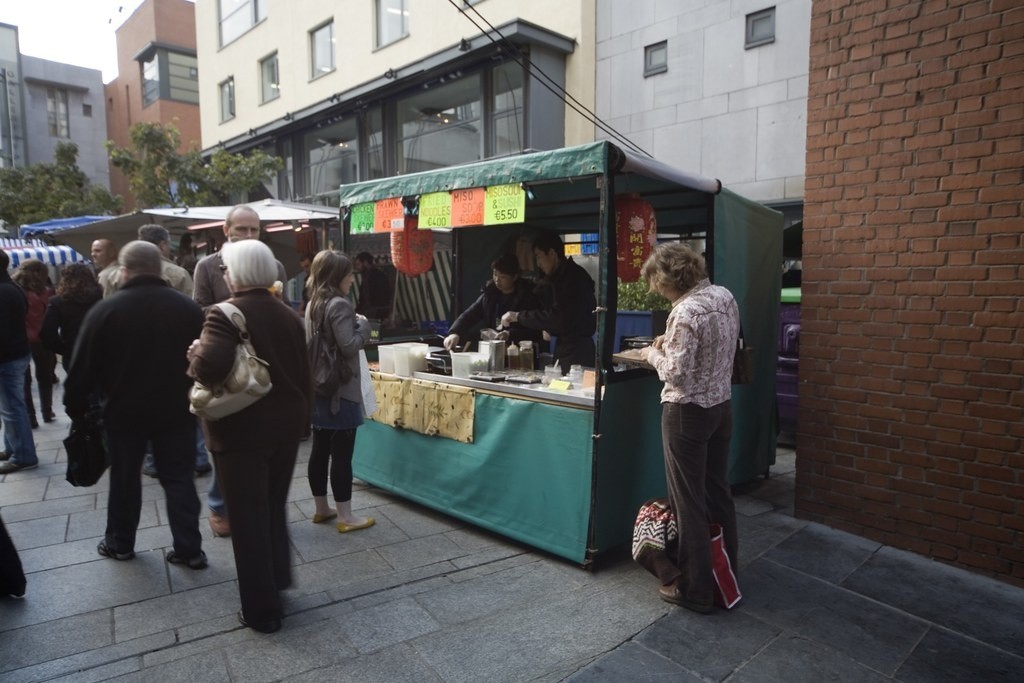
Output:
[62,401,111,488]
[302,296,350,400]
[631,497,743,610]
[188,303,273,419]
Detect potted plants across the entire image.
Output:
[549,275,672,354]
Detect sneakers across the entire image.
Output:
[142,467,159,478]
[208,511,230,535]
[193,465,212,478]
[166,550,208,569]
[0,458,39,474]
[97,538,135,561]
[0,451,12,460]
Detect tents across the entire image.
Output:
[19,197,341,263]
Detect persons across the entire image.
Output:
[304,250,380,533]
[64,239,212,570]
[193,207,288,535]
[442,233,597,375]
[186,238,306,632]
[638,241,742,612]
[0,224,193,474]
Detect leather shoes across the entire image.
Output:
[658,584,714,613]
[236,608,281,633]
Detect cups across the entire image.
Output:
[544,364,561,379]
[273,280,283,300]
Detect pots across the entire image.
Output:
[429,347,468,376]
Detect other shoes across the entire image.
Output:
[336,516,375,533]
[43,412,56,422]
[31,417,38,429]
[312,509,336,521]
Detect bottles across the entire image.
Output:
[519,340,534,371]
[507,341,519,370]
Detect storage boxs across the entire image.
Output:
[377,344,394,375]
[450,352,489,380]
[391,342,429,378]
[477,339,506,371]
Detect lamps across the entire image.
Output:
[384,67,397,79]
[327,93,340,103]
[458,37,471,52]
[216,140,225,147]
[282,112,294,121]
[247,128,257,136]
